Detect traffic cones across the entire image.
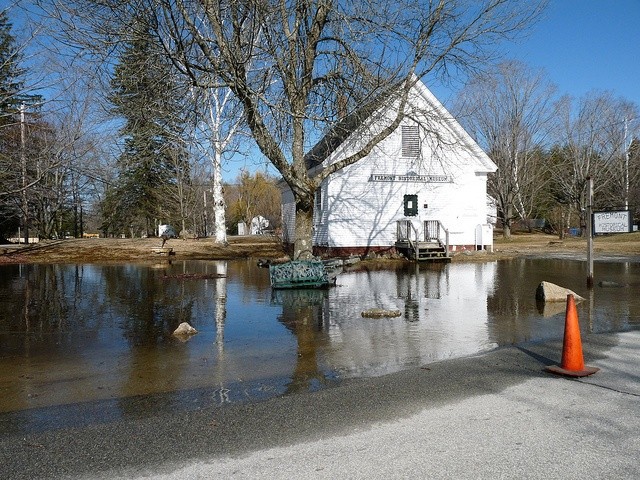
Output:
[545,294,600,377]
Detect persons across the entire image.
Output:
[160,231,168,248]
